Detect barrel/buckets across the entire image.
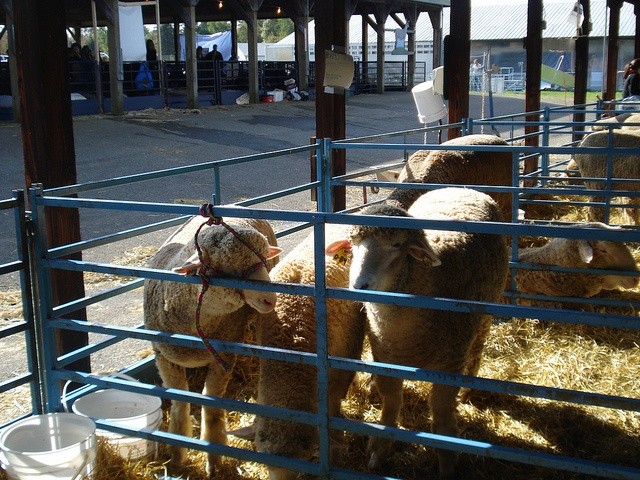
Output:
[0,412,96,480]
[274,89,283,101]
[412,80,448,124]
[72,387,163,466]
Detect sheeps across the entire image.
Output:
[231,201,366,479]
[142,203,283,480]
[512,221,640,298]
[380,132,518,204]
[571,132,640,219]
[322,183,511,480]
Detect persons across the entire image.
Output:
[204,44,223,60]
[80,44,90,55]
[470,59,485,89]
[621,57,640,97]
[145,40,159,69]
[228,51,238,61]
[196,46,204,60]
[69,42,90,62]
